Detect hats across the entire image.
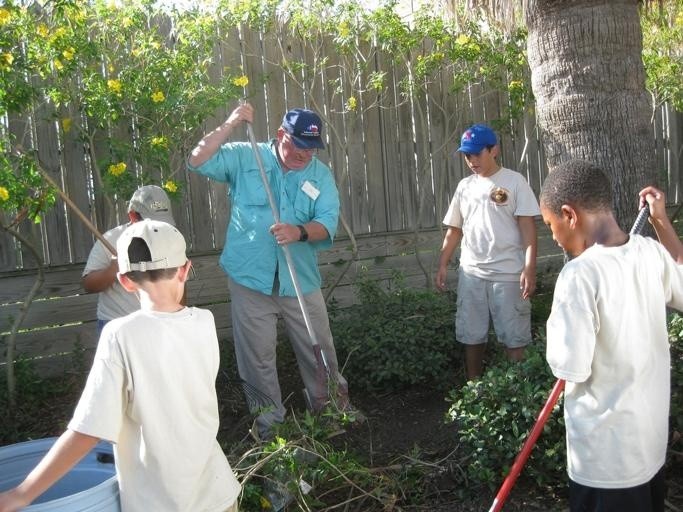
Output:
[117,184,186,274]
[455,126,496,153]
[282,109,325,149]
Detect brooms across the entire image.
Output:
[242,109,367,434]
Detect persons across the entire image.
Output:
[80,183,178,344]
[434,123,541,380]
[186,101,362,443]
[541,157,682,504]
[1,217,247,512]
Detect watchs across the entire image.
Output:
[297,225,308,241]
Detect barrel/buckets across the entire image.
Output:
[0,432,120,511]
